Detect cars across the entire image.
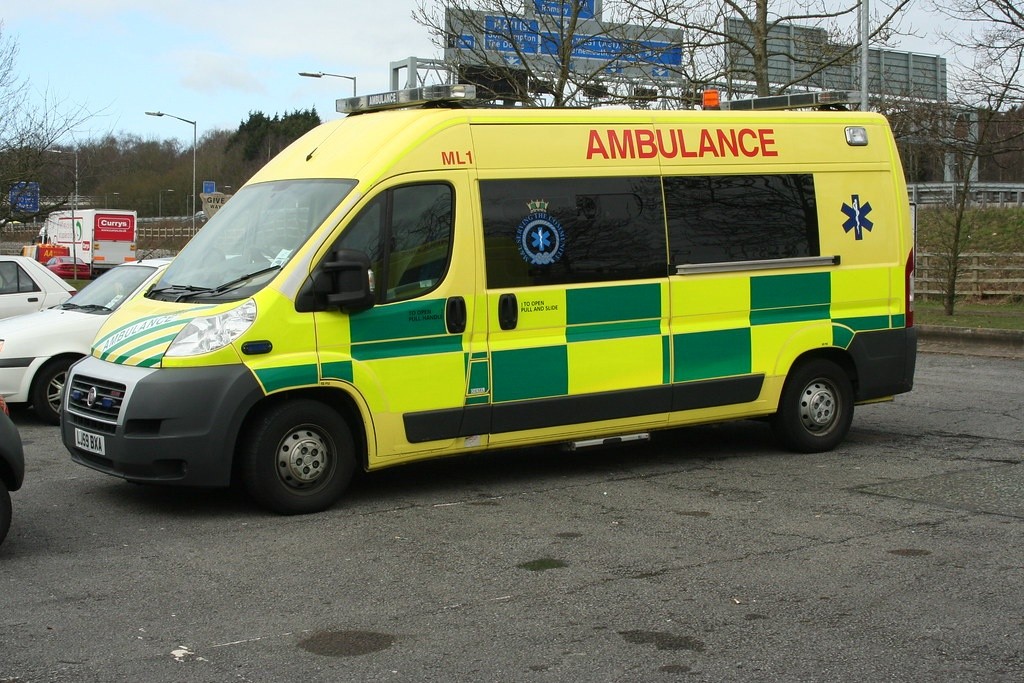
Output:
[39,254,90,281]
[0,254,80,322]
[0,255,242,427]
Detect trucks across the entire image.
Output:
[28,208,138,276]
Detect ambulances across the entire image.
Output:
[59,88,919,516]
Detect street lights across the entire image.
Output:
[47,149,78,212]
[300,71,356,99]
[185,194,194,219]
[159,188,175,220]
[144,111,197,238]
[104,192,121,211]
